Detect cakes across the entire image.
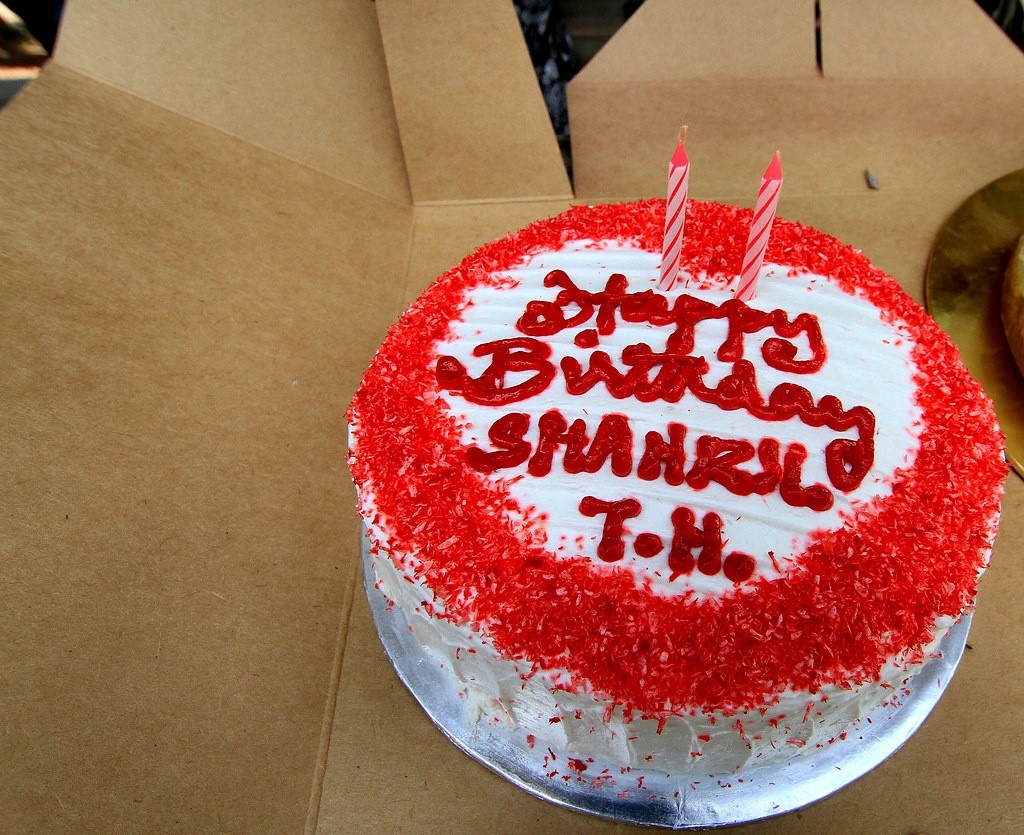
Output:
[346,199,1008,771]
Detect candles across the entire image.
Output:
[733,150,784,301]
[657,126,691,294]
